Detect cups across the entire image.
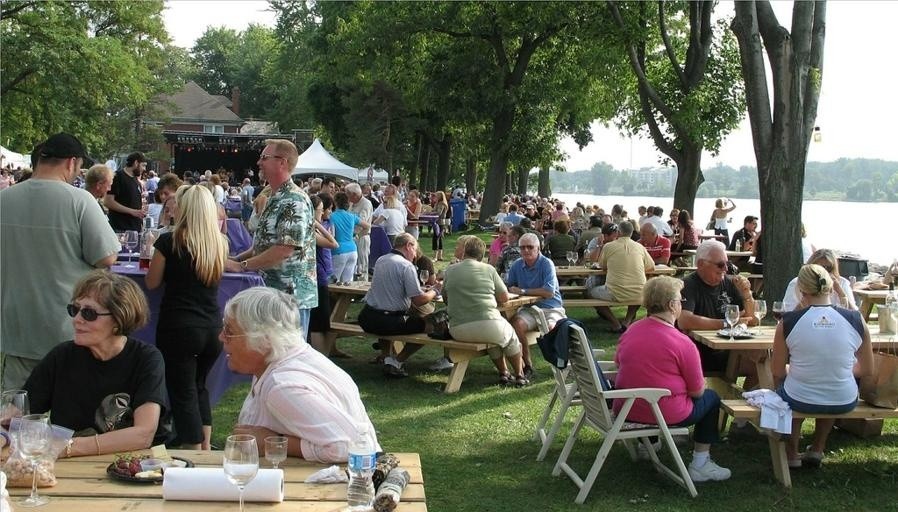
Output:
[876,307,890,333]
[139,458,163,471]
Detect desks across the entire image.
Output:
[329,280,544,378]
[487,229,896,329]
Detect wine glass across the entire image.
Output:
[724,303,742,342]
[419,268,430,288]
[264,435,288,467]
[354,263,368,287]
[222,433,260,511]
[750,298,769,336]
[771,300,785,324]
[0,389,32,434]
[566,251,578,269]
[121,228,139,269]
[19,414,55,507]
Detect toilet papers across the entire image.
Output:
[161,466,285,504]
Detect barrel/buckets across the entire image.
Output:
[877,304,898,334]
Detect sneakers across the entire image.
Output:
[382,354,408,377]
[429,358,454,369]
[638,439,662,460]
[688,461,731,482]
[729,421,759,443]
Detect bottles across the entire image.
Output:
[346,421,378,508]
[885,280,897,307]
[582,239,591,268]
[138,215,158,269]
[735,239,740,252]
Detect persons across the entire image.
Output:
[773,264,876,467]
[358,222,567,385]
[615,275,742,482]
[675,240,759,441]
[782,248,859,438]
[2,131,482,461]
[495,195,758,332]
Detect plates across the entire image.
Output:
[718,328,753,338]
[106,454,194,483]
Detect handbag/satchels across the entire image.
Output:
[425,310,450,340]
[859,350,898,409]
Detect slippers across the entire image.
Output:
[789,444,824,469]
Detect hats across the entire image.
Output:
[41,132,96,163]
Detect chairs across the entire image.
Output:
[549,324,698,506]
[527,303,619,463]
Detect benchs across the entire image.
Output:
[716,397,897,486]
[330,321,547,390]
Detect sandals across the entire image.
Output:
[498,365,534,386]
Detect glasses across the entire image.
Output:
[261,154,282,160]
[708,260,725,267]
[221,325,246,339]
[67,304,111,321]
[520,246,534,250]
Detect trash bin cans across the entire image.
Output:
[449,199,469,233]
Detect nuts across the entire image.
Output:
[4,459,54,487]
